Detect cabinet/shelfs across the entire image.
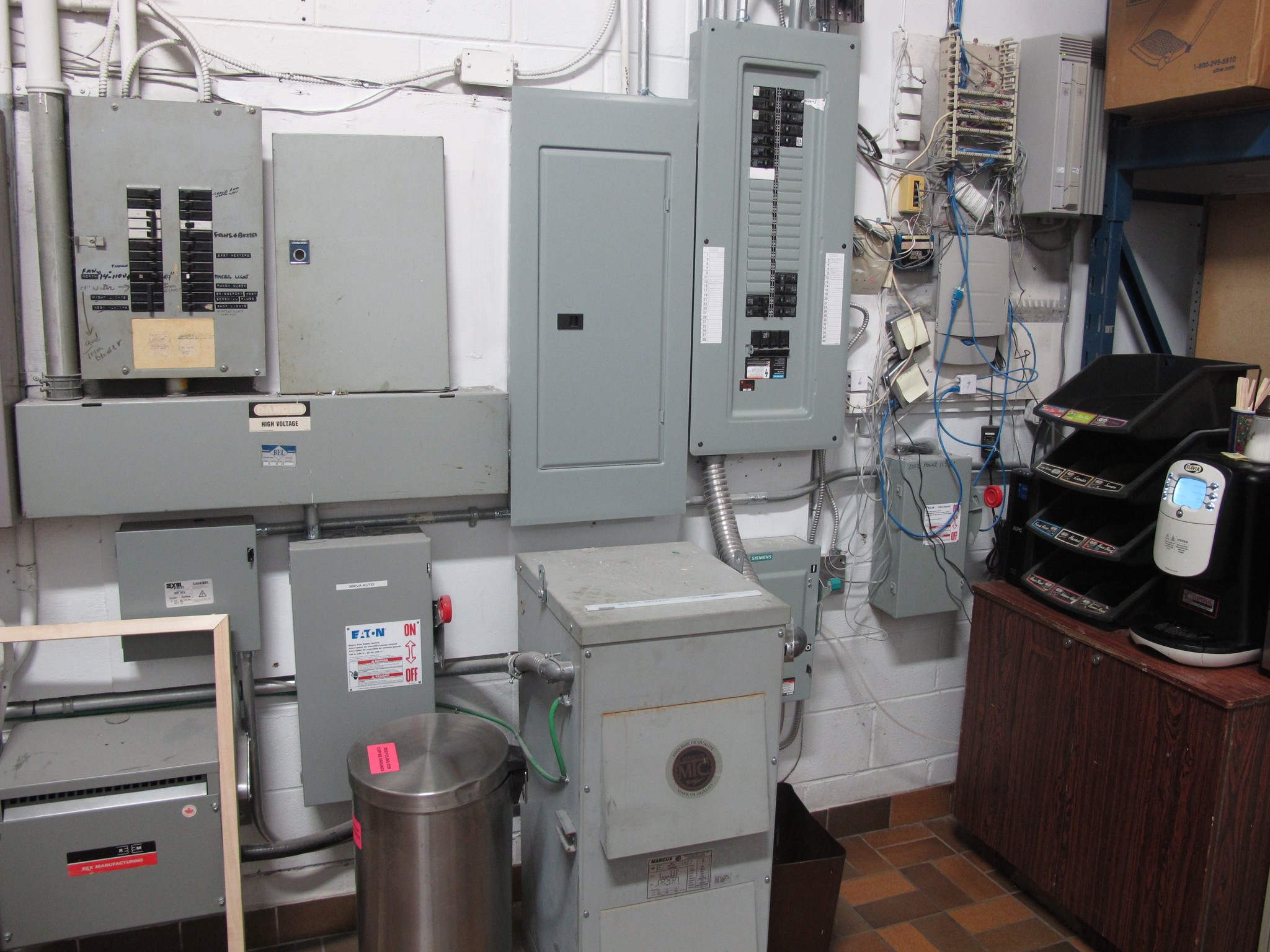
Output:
[954,592,1270,952]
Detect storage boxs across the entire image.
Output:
[1102,0,1270,117]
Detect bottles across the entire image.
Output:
[1243,408,1270,464]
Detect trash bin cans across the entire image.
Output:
[346,712,513,952]
[768,781,846,952]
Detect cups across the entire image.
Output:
[1230,407,1256,454]
